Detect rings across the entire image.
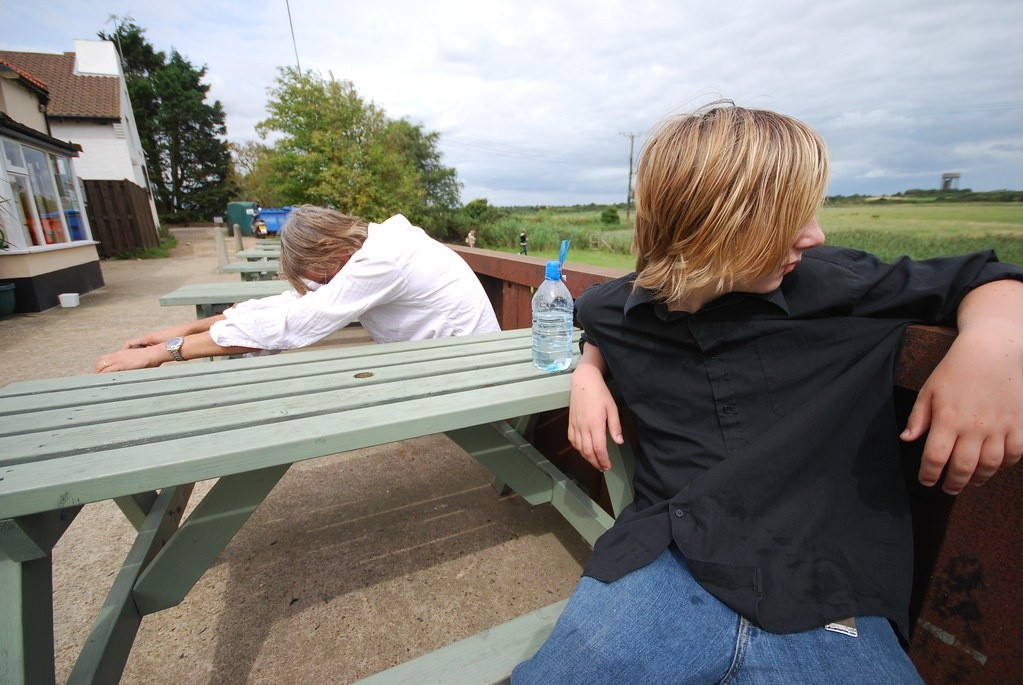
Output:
[104,361,106,367]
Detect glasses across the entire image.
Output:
[321,270,330,286]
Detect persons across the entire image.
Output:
[468,229,477,248]
[518,229,528,255]
[511,98,1023,685]
[93,205,502,374]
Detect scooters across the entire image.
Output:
[250,206,268,240]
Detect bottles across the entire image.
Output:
[531,262,575,372]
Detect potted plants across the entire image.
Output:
[0,180,15,321]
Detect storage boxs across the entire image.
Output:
[58,293,80,308]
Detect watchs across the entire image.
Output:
[164,336,185,361]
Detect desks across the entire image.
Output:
[254,245,281,250]
[236,251,280,259]
[0,326,635,685]
[256,242,281,244]
[223,261,280,281]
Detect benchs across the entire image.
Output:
[348,597,572,685]
[159,279,361,362]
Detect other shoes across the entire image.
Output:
[518,252,520,255]
